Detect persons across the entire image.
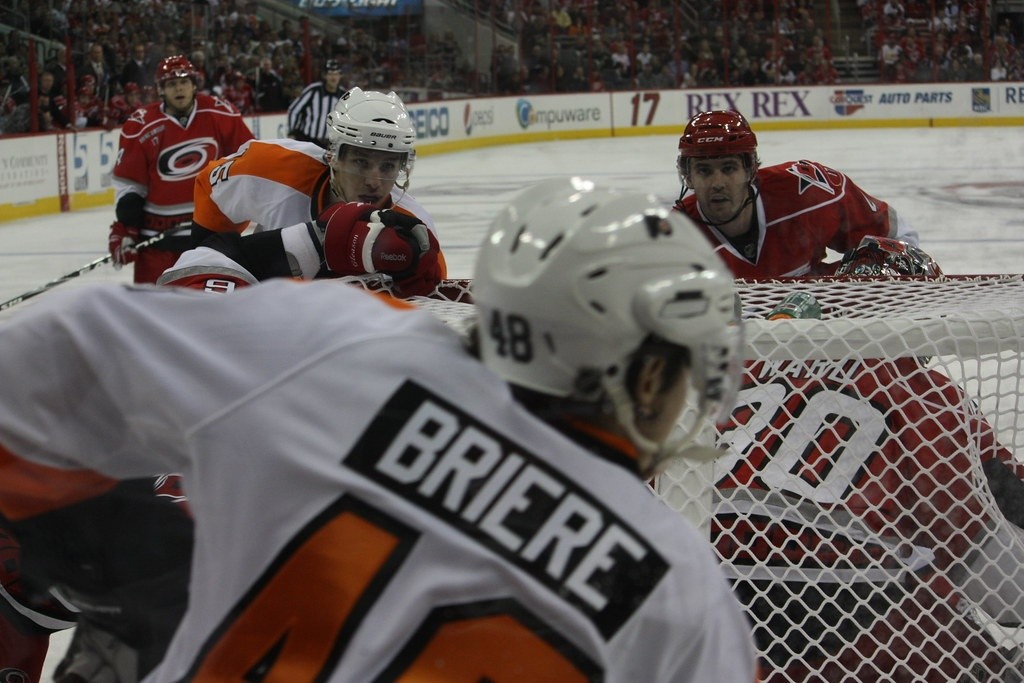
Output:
[672,110,920,283]
[711,235,1023,683]
[0,177,761,682]
[109,56,256,284]
[286,58,347,150]
[0,0,1024,135]
[52,85,444,683]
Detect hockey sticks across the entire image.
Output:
[0,221,195,312]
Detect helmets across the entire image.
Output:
[832,235,943,282]
[156,55,196,82]
[678,110,756,179]
[325,59,341,73]
[474,176,736,404]
[325,87,416,166]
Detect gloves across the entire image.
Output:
[317,200,441,300]
[109,224,139,269]
[0,475,196,682]
